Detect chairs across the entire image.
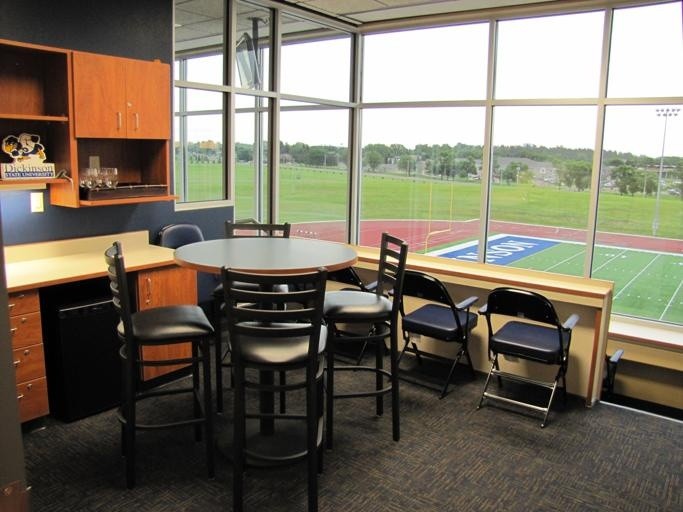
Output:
[323,266,397,372]
[103,241,217,484]
[475,288,579,427]
[314,230,412,453]
[157,222,224,303]
[218,218,290,416]
[219,264,328,512]
[390,268,480,401]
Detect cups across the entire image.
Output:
[79,166,118,190]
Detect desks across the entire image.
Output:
[325,240,615,409]
[172,237,358,481]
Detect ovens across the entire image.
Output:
[39,272,135,424]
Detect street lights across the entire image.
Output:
[652,104,678,237]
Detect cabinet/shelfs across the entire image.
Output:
[45,49,178,209]
[3,229,198,426]
[0,38,72,186]
